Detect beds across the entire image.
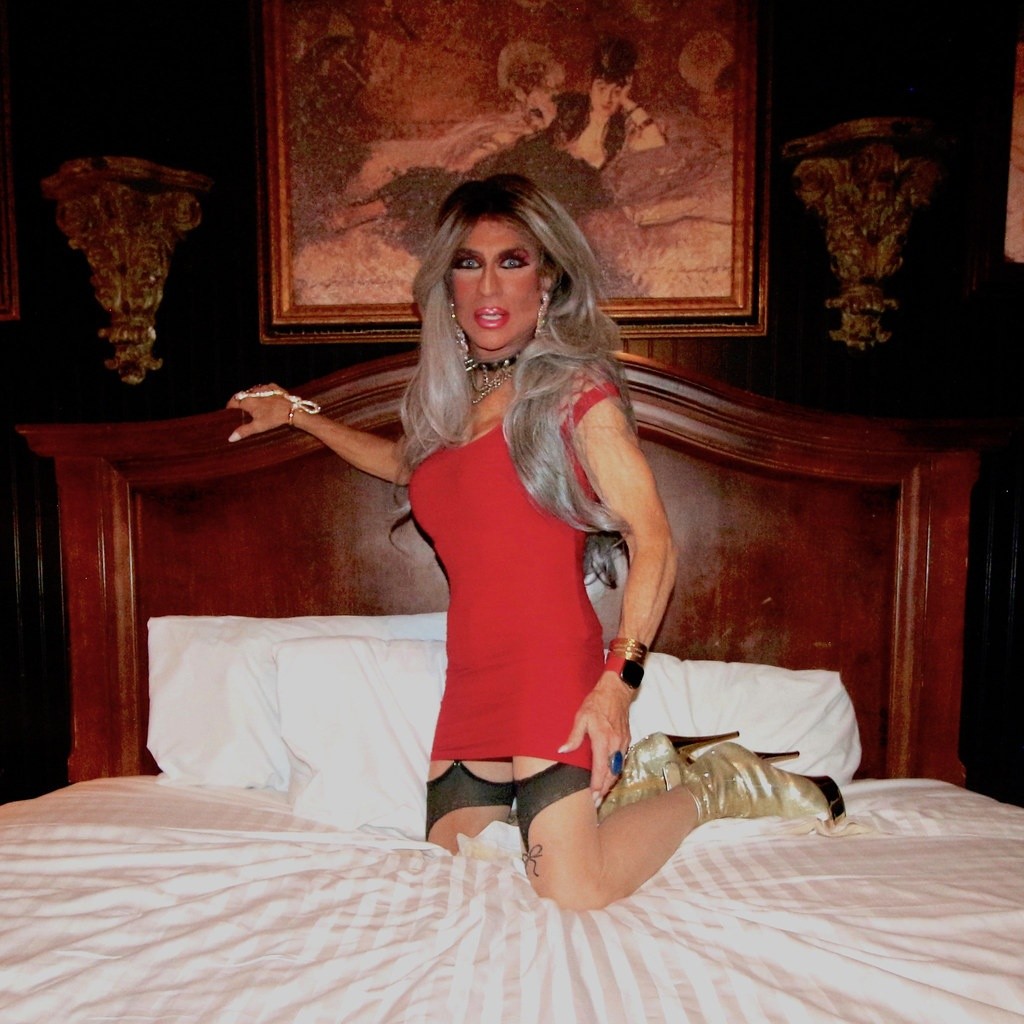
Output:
[0,347,1024,1023]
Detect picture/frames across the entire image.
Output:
[249,1,771,348]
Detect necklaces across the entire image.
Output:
[462,352,522,404]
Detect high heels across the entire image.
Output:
[597,732,744,824]
[680,741,845,828]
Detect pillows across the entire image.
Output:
[601,649,861,787]
[147,613,450,792]
[273,637,452,837]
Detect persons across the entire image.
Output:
[225,173,848,913]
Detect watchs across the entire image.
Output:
[605,652,645,690]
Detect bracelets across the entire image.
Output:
[233,389,321,425]
[608,637,647,665]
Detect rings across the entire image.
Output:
[607,751,624,776]
